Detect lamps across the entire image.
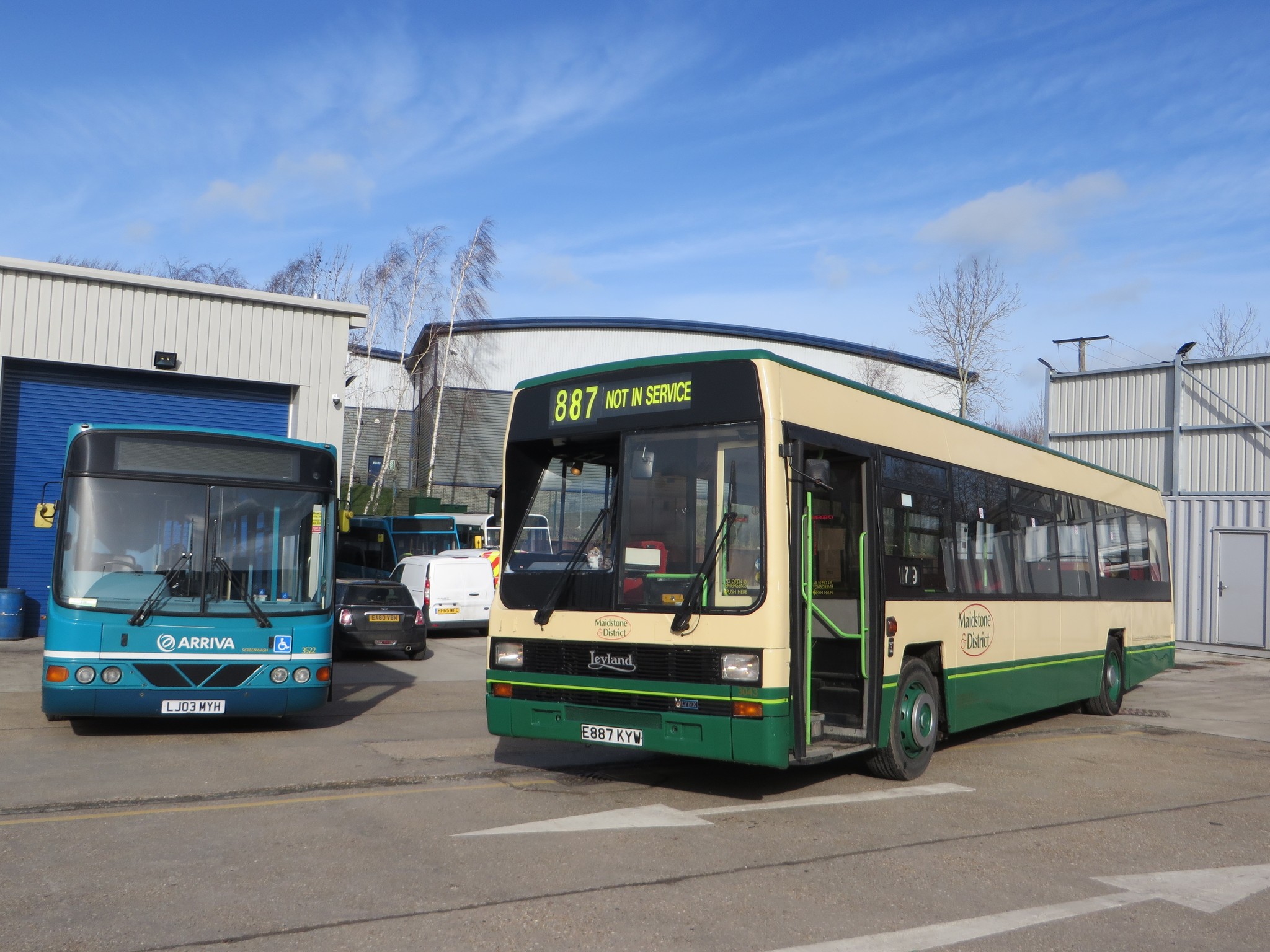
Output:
[346,376,357,387]
[1176,342,1197,356]
[153,351,177,370]
[1037,358,1054,370]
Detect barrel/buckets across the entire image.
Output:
[0,587,27,640]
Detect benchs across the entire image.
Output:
[1038,554,1148,579]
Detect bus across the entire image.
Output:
[485,349,1177,782]
[334,513,554,662]
[34,423,353,718]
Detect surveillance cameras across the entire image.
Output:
[450,347,457,352]
[332,397,340,405]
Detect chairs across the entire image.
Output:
[622,541,667,604]
[164,543,188,565]
[86,554,136,573]
